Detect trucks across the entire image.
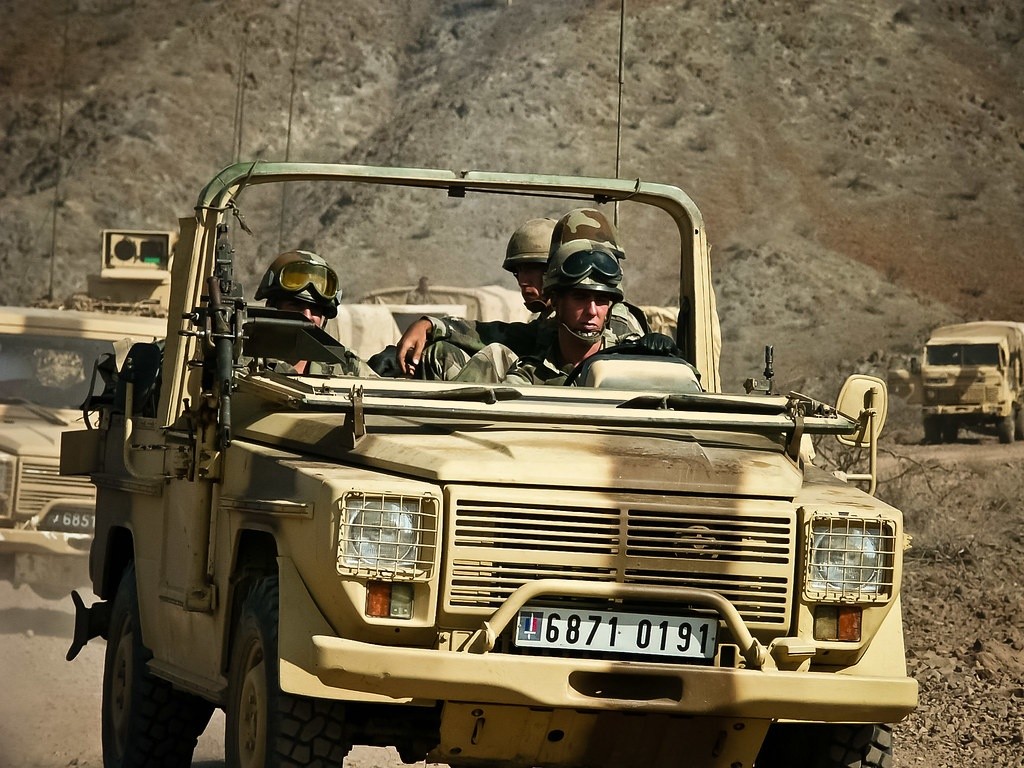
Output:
[910,320,1024,445]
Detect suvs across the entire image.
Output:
[1,301,173,602]
[59,162,921,768]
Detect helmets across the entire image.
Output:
[542,239,624,301]
[502,216,559,269]
[253,250,343,319]
[549,208,626,261]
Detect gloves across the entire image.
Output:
[634,333,677,357]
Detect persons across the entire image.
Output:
[501,218,559,323]
[503,239,682,387]
[238,251,380,377]
[396,208,656,384]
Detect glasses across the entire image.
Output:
[278,259,340,301]
[561,249,621,279]
